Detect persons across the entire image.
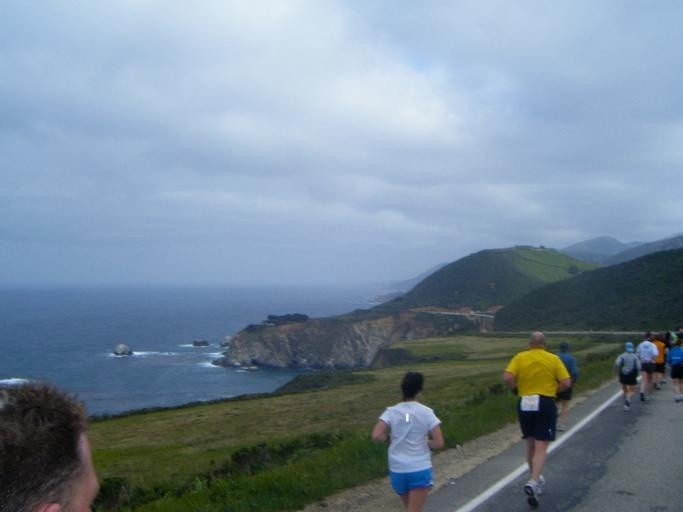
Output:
[500,329,569,511]
[366,368,444,512]
[553,338,578,433]
[0,377,101,511]
[612,324,682,412]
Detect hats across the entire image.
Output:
[625,343,633,352]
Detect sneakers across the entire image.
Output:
[639,392,645,401]
[524,475,544,507]
[556,423,566,431]
[623,399,630,411]
[674,394,683,402]
